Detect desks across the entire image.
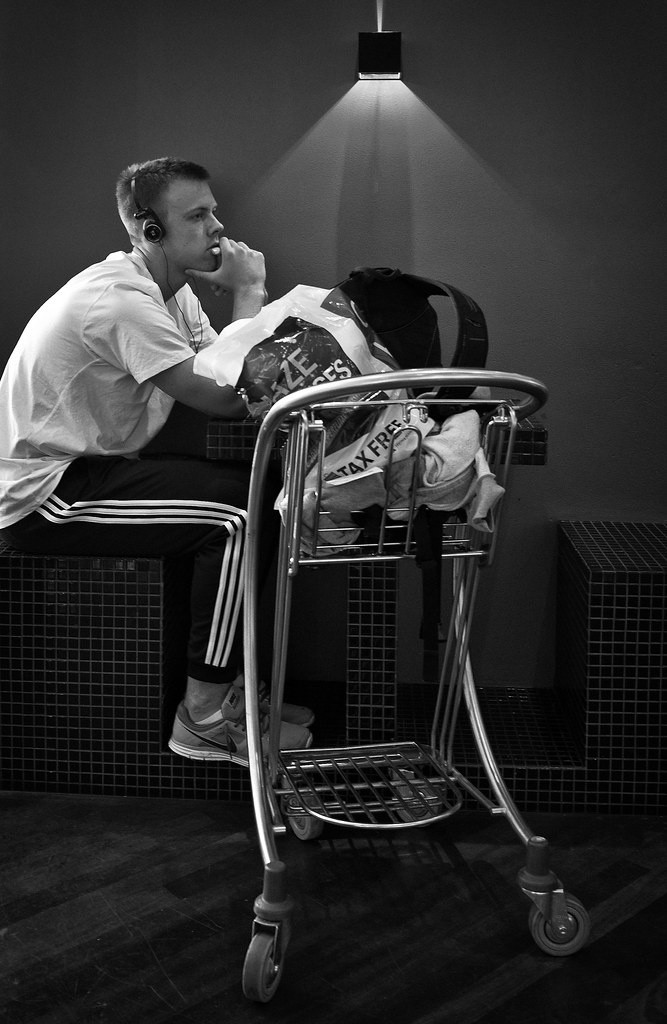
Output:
[207,399,548,745]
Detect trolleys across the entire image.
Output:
[242,366,596,1005]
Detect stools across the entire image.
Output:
[552,520,667,766]
[0,532,175,754]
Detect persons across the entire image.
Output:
[0,157,316,769]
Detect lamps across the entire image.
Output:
[359,32,402,80]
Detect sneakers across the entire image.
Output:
[167,673,313,768]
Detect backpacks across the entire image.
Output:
[338,265,490,422]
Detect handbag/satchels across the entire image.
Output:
[192,283,436,515]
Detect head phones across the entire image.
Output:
[129,167,166,244]
